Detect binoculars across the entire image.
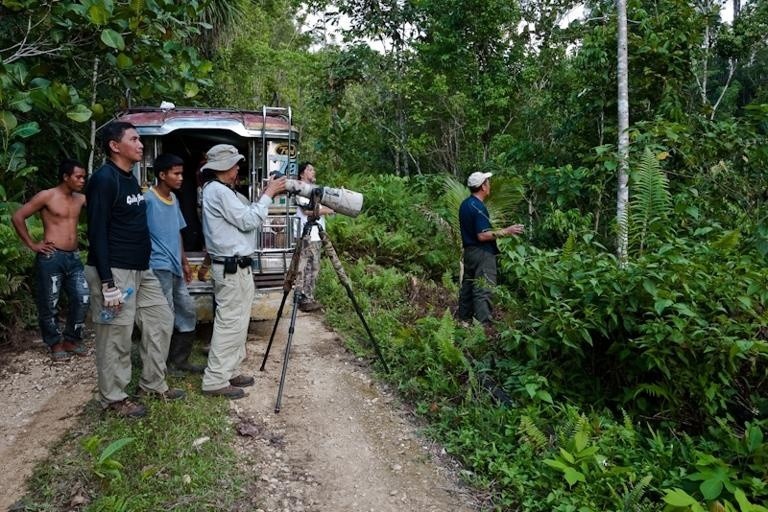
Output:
[269,170,364,222]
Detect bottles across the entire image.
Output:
[99,287,134,321]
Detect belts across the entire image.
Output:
[210,257,226,265]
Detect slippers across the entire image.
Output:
[66,347,90,357]
[52,352,70,361]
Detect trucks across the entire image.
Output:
[93,90,302,325]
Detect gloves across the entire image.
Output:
[102,282,125,307]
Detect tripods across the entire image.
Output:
[260,221,390,414]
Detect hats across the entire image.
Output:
[200,144,246,173]
[467,171,493,188]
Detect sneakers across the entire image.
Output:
[109,397,146,418]
[170,362,205,375]
[229,375,254,387]
[135,385,185,402]
[202,384,244,400]
[298,296,322,313]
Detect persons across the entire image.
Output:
[292,161,334,310]
[10,158,91,363]
[199,143,287,400]
[84,122,185,419]
[457,171,525,323]
[141,153,207,380]
